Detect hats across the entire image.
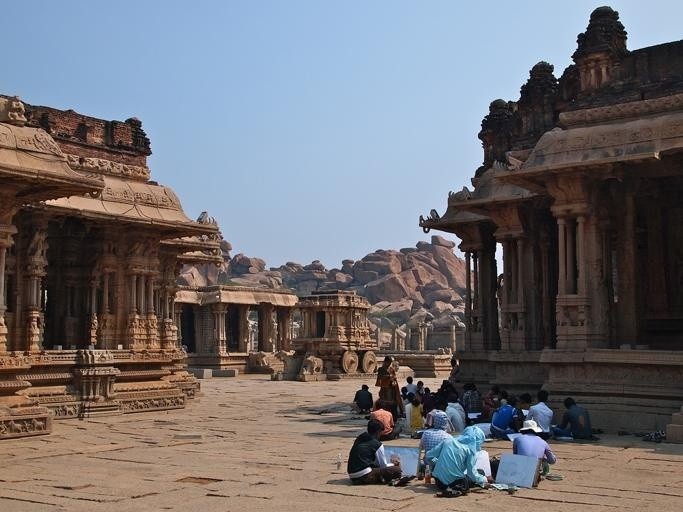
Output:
[518,420,542,433]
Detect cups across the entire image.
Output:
[508,484,514,495]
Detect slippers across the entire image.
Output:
[436,487,463,498]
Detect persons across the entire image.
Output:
[375,355,404,419]
[347,376,592,498]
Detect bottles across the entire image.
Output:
[424,464,431,485]
[336,453,344,473]
[419,459,425,477]
